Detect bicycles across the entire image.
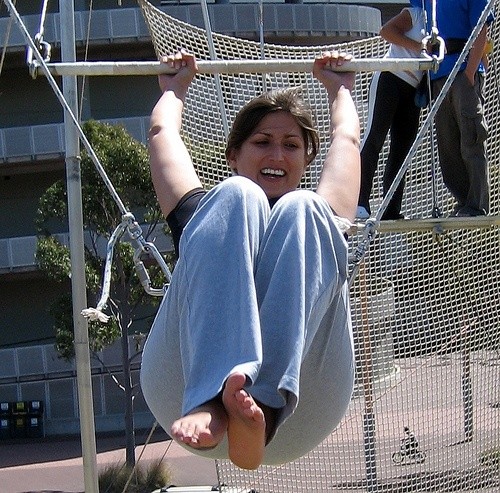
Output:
[391,425,427,466]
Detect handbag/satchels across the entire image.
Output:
[415,72,430,107]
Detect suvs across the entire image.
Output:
[150,483,257,493]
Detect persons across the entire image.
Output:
[407,0,495,216]
[356,6,489,219]
[401,426,418,458]
[140,48,362,471]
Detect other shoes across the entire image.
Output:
[447,213,471,217]
[356,206,370,218]
